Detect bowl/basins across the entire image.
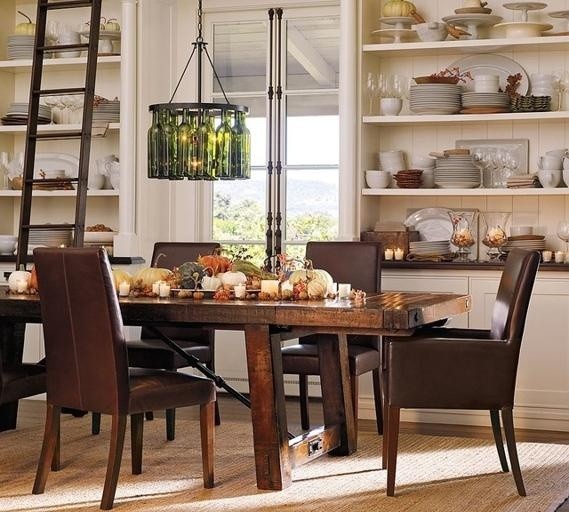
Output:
[70,181,78,190]
[0,234,18,255]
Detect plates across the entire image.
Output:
[433,154,481,189]
[461,92,511,107]
[501,239,547,253]
[7,102,52,120]
[499,253,543,263]
[28,223,75,246]
[508,95,552,112]
[98,53,121,56]
[22,153,79,179]
[229,290,262,297]
[196,289,216,299]
[455,7,492,14]
[444,54,530,96]
[7,34,53,60]
[409,84,464,114]
[170,288,196,298]
[548,9,569,31]
[393,170,424,188]
[92,101,120,123]
[542,32,569,36]
[83,243,113,246]
[402,207,469,255]
[541,31,569,36]
[79,30,121,52]
[412,77,462,84]
[72,230,119,243]
[492,22,553,40]
[0,114,55,126]
[504,235,545,241]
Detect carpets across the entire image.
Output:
[0,410,569,512]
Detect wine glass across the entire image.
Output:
[364,72,409,116]
[470,147,522,188]
[551,70,569,111]
[557,220,569,253]
[46,20,64,60]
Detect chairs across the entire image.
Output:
[382,248,543,497]
[280,243,384,445]
[31,245,215,507]
[124,241,221,428]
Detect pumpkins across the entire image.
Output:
[7,264,32,293]
[110,267,133,292]
[220,270,246,288]
[133,266,172,290]
[175,262,209,287]
[288,267,334,289]
[29,265,39,290]
[198,253,231,275]
[381,0,415,16]
[307,277,327,297]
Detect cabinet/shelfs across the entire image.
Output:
[356,0,568,271]
[0,1,136,263]
[1,263,129,399]
[352,274,568,433]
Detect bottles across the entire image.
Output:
[197,105,215,178]
[170,112,184,180]
[163,109,177,178]
[216,107,232,178]
[187,113,199,177]
[220,111,235,181]
[208,113,216,142]
[148,109,165,177]
[157,111,164,129]
[242,113,251,179]
[231,110,244,179]
[178,108,191,176]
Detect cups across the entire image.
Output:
[48,169,65,178]
[537,155,562,170]
[416,21,449,42]
[538,169,562,188]
[562,170,569,188]
[366,170,392,188]
[87,154,118,190]
[474,74,499,93]
[380,98,402,116]
[545,148,568,157]
[510,226,533,237]
[110,161,120,190]
[410,156,435,188]
[377,149,407,188]
[531,73,559,110]
[562,156,569,170]
[54,31,81,58]
[480,211,512,265]
[447,210,481,262]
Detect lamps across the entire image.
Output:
[146,1,252,182]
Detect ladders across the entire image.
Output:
[14,1,102,427]
